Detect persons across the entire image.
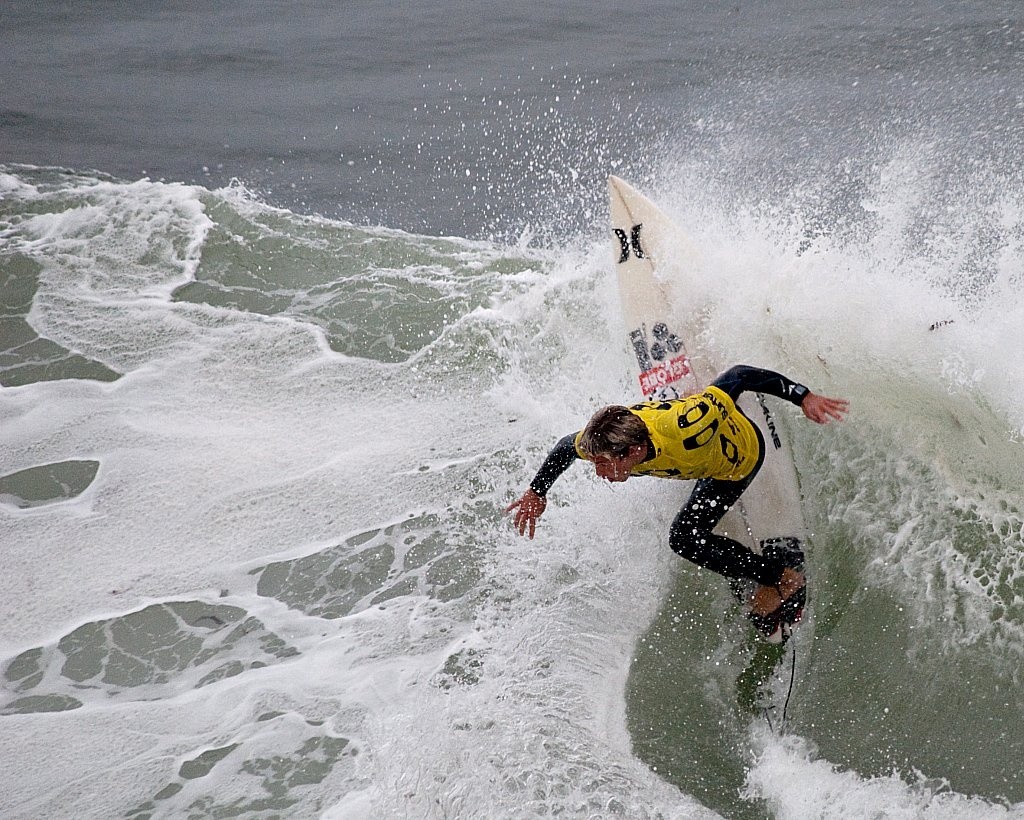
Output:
[506,364,849,617]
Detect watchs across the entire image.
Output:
[791,383,810,406]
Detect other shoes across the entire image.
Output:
[745,567,803,619]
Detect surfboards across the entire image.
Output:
[605,171,816,742]
